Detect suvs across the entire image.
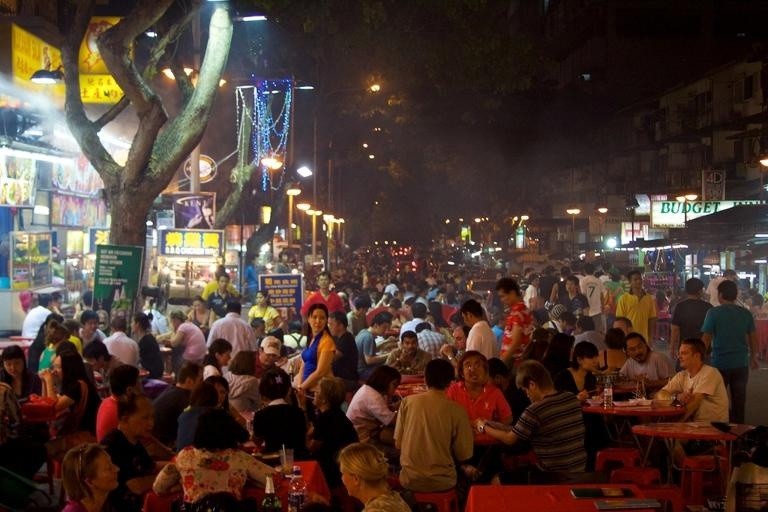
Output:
[395,255,416,273]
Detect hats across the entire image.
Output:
[260,336,282,357]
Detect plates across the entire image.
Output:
[614,399,651,406]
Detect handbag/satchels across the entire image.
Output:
[63,405,85,430]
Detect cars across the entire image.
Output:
[169,276,206,304]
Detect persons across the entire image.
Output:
[1,241,767,512]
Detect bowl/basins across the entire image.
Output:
[586,398,603,407]
[657,397,673,406]
[262,455,280,466]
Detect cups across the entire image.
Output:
[279,450,294,474]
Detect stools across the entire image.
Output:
[595,447,641,471]
[678,454,727,503]
[635,483,684,512]
[607,466,660,486]
[632,422,755,481]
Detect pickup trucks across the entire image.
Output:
[523,260,572,272]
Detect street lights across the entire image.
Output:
[624,198,640,241]
[675,194,698,228]
[338,155,374,211]
[235,83,311,234]
[310,84,380,260]
[285,184,301,266]
[326,143,369,212]
[474,217,489,241]
[566,205,581,259]
[513,215,529,254]
[594,205,609,249]
[296,203,345,270]
[758,157,768,204]
[261,152,283,260]
[445,218,464,240]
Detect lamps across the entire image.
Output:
[296,81,317,89]
[235,5,268,23]
[30,65,64,86]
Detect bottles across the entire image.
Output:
[109,284,132,328]
[261,472,283,512]
[252,447,262,461]
[293,465,301,476]
[603,378,614,409]
[287,471,306,512]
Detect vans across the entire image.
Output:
[579,242,606,251]
